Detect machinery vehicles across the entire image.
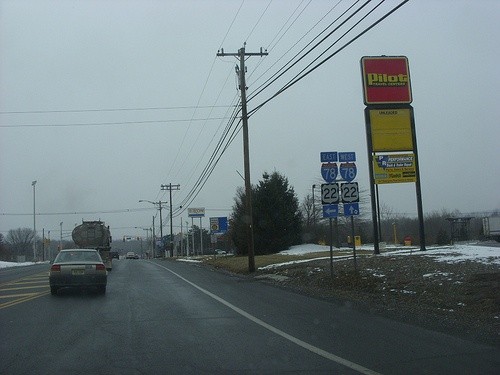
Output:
[71,218,113,272]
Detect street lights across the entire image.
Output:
[59,221,64,249]
[138,199,163,259]
[32,180,37,263]
[134,227,149,251]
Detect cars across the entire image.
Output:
[111,251,119,260]
[126,251,140,260]
[49,248,107,295]
